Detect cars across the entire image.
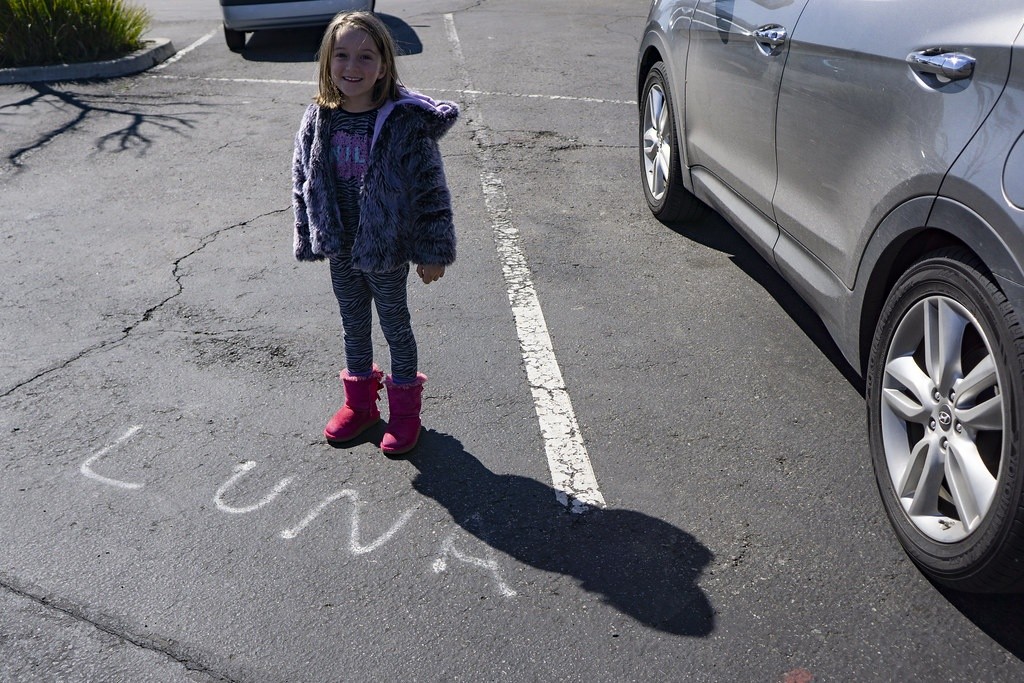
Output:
[218,0,381,52]
[635,0,1024,595]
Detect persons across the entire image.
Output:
[291,12,459,457]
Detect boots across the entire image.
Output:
[381,372,426,454]
[324,362,384,443]
